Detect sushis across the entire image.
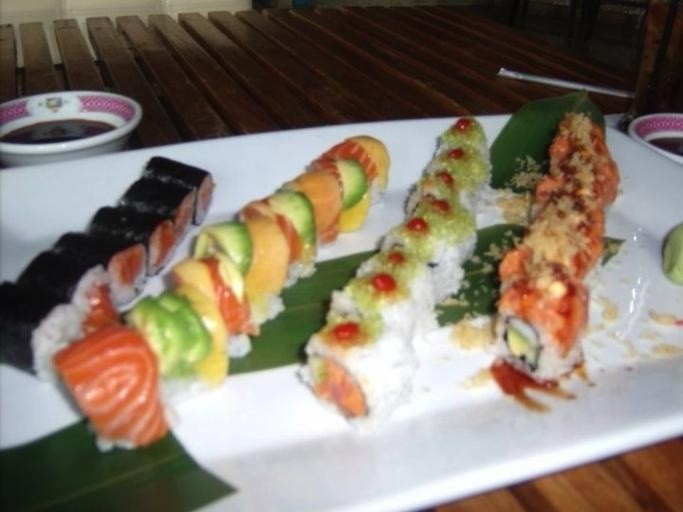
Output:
[0,117,622,453]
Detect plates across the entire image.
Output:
[625,113,683,161]
[0,114,683,511]
[0,90,144,165]
